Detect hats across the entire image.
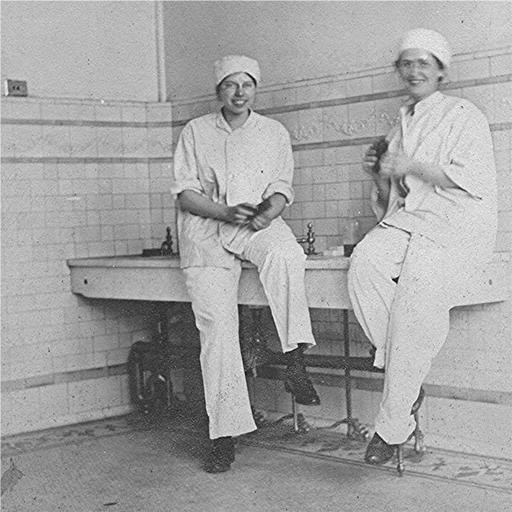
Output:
[210,55,261,86]
[396,27,450,72]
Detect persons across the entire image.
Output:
[167,54,318,473]
[343,27,499,465]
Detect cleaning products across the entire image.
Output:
[342,204,362,255]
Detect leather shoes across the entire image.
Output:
[410,384,426,415]
[206,441,236,474]
[364,416,418,467]
[283,366,323,406]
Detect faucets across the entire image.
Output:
[297,222,316,255]
[160,226,172,254]
